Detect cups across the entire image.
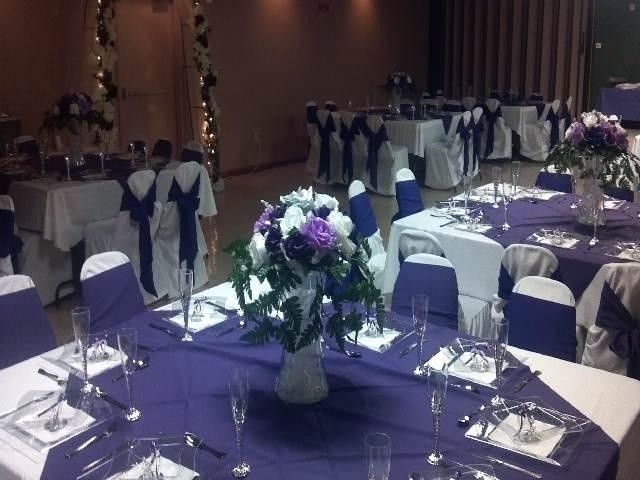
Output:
[362,430,391,478]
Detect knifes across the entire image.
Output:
[513,369,541,393]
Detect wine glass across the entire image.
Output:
[225,366,251,477]
[490,318,510,401]
[34,133,151,180]
[346,93,439,121]
[461,159,521,233]
[410,293,429,378]
[177,268,193,342]
[117,327,143,423]
[70,305,96,394]
[423,362,450,466]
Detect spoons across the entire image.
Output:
[455,405,484,425]
[215,318,248,337]
[64,421,117,459]
[184,432,224,460]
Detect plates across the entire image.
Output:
[522,227,638,261]
[423,335,530,388]
[343,315,419,359]
[100,433,200,480]
[462,395,594,470]
[0,390,120,465]
[160,294,231,322]
[404,461,497,479]
[40,337,130,382]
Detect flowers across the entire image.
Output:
[90,0,224,195]
[33,91,116,143]
[383,68,416,90]
